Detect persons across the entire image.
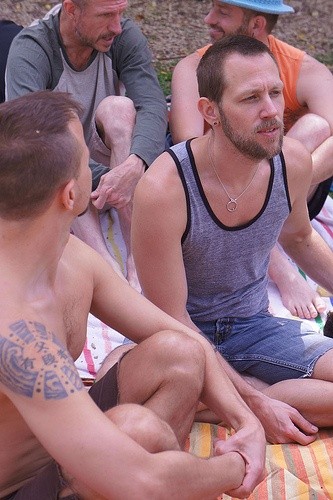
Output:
[94,35,333,446]
[4,0,168,294]
[0,89,267,500]
[171,0,333,319]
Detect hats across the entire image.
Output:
[220,0,295,13]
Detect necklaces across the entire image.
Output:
[207,133,260,212]
